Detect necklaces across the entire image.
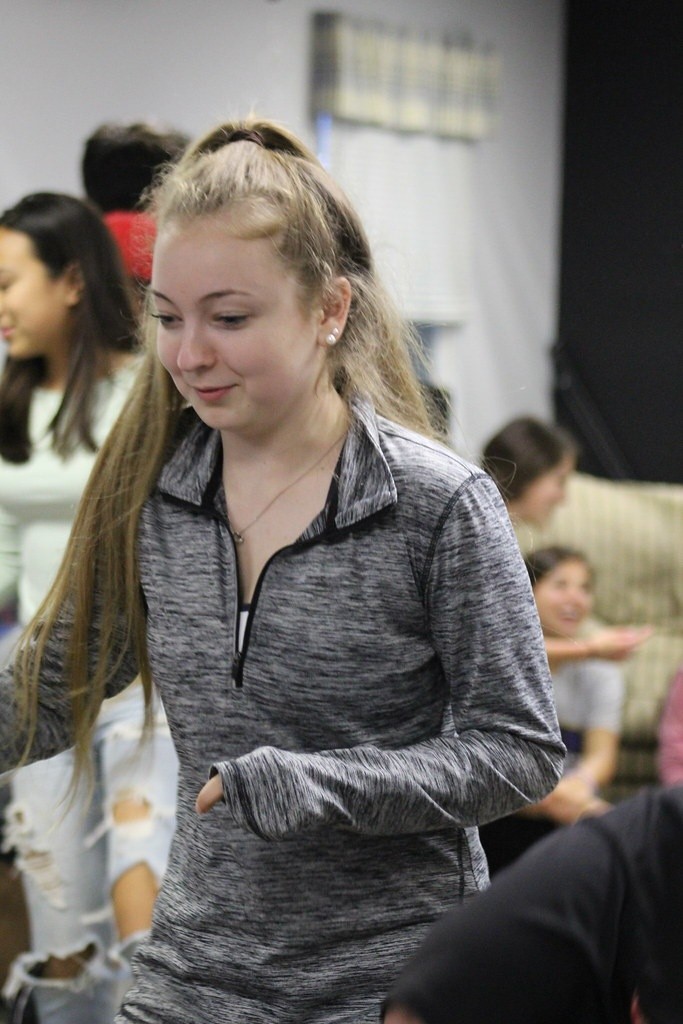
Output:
[224,430,347,544]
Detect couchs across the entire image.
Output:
[518,468,683,738]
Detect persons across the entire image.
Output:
[80,124,192,301]
[1,123,566,1024]
[477,414,575,546]
[0,193,180,1023]
[477,546,627,884]
[378,785,683,1024]
